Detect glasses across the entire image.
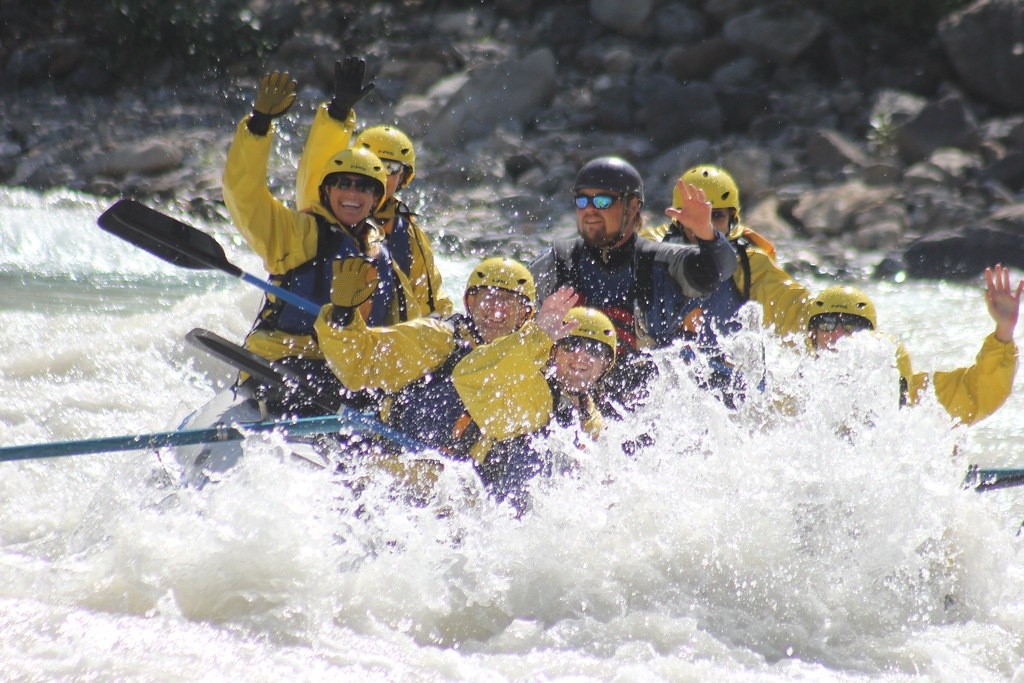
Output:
[574,194,624,208]
[815,314,864,335]
[709,209,732,223]
[329,175,377,194]
[383,161,403,176]
[560,337,608,359]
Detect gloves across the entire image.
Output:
[247,70,298,137]
[329,256,378,324]
[325,56,376,122]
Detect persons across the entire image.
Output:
[222,58,453,419]
[807,262,1024,427]
[527,155,776,409]
[313,253,640,523]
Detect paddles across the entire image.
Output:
[962,467,1024,493]
[96,199,321,317]
[0,414,375,463]
[183,327,426,454]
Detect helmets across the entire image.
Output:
[463,257,535,322]
[317,148,386,217]
[562,306,616,379]
[672,165,738,219]
[576,156,644,203]
[351,125,416,189]
[803,285,877,336]
